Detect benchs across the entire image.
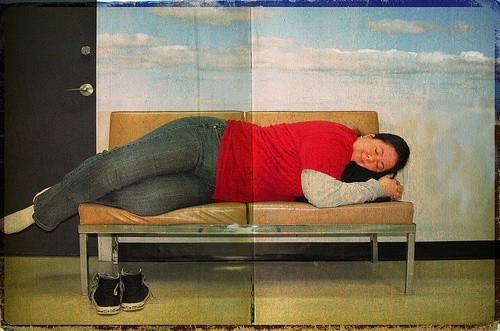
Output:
[78,110,418,298]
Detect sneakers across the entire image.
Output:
[88,272,121,315]
[121,268,159,311]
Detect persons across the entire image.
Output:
[0,115,411,234]
